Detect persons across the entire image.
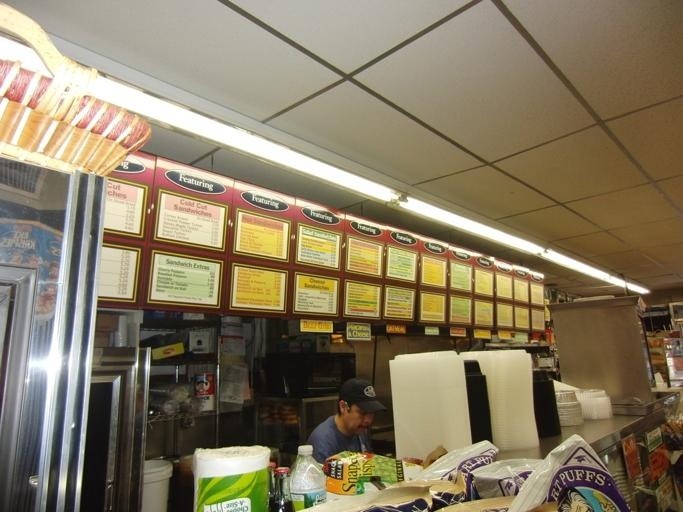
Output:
[306,375,388,466]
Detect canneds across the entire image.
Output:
[194,395,216,412]
[193,373,215,397]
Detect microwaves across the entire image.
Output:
[265,351,357,397]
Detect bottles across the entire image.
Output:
[193,363,214,411]
[269,445,326,512]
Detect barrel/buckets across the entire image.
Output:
[141,459,174,512]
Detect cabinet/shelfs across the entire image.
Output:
[140,311,257,466]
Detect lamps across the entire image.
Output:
[545,250,650,297]
[391,189,544,258]
[2,35,401,209]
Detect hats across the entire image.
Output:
[339,377,387,413]
